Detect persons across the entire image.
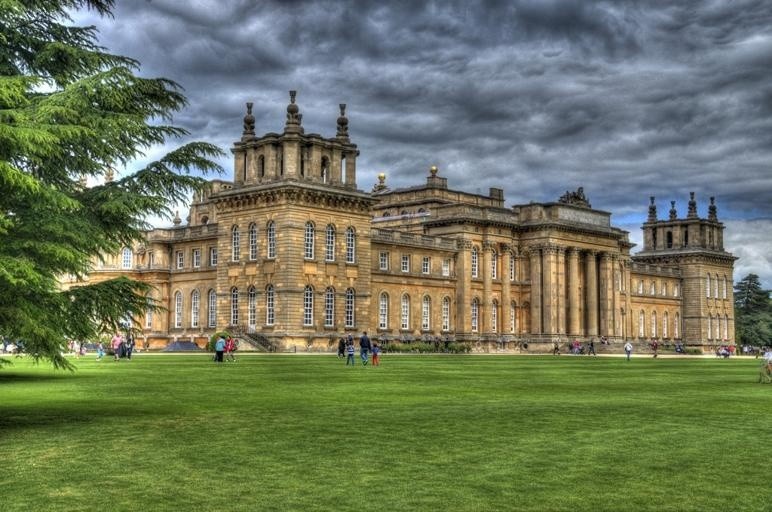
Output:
[716,343,772,373]
[337,331,380,367]
[652,339,658,358]
[212,335,236,362]
[96,328,149,362]
[552,336,609,356]
[624,341,632,359]
[433,335,450,353]
[80,343,87,356]
